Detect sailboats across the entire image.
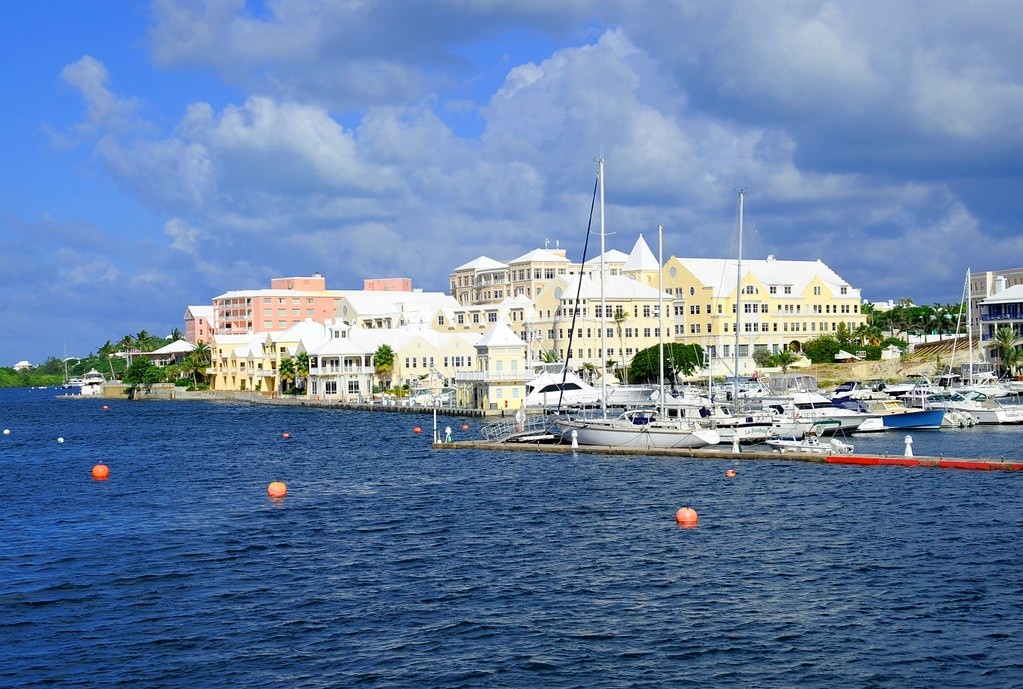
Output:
[520,154,1023,449]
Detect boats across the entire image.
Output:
[67,378,84,388]
[762,438,854,457]
[81,367,106,390]
